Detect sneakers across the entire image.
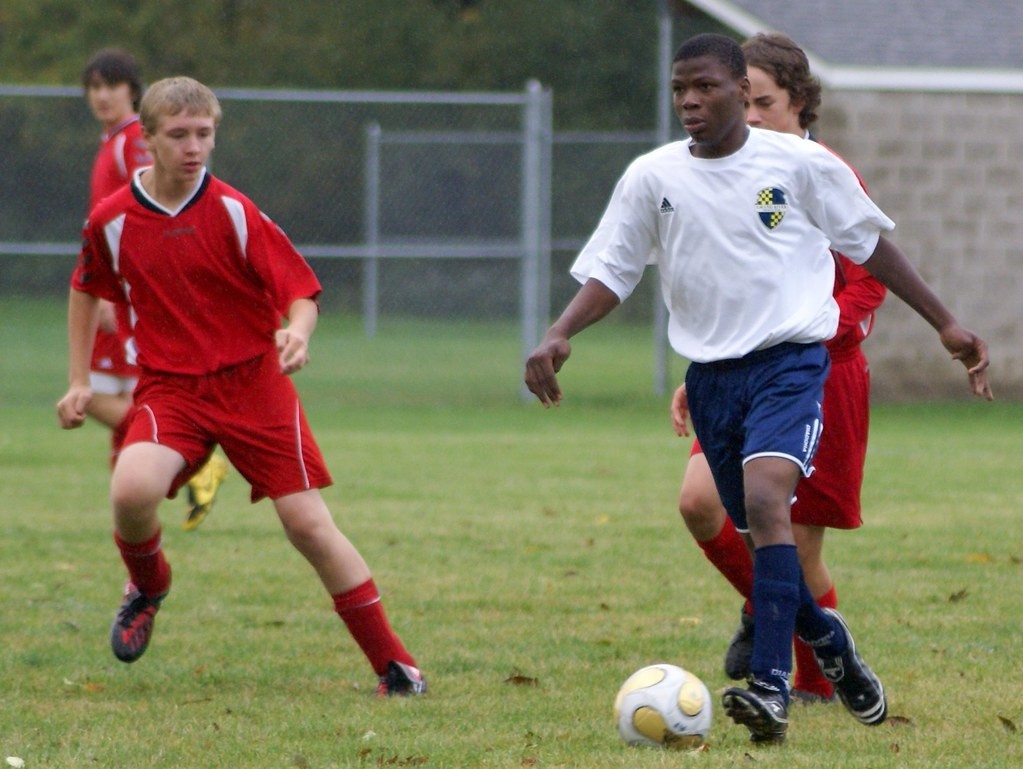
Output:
[377,659,424,702]
[808,610,886,725]
[721,674,790,745]
[181,452,230,533]
[789,684,838,708]
[112,567,173,663]
[723,604,760,679]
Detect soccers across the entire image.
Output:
[611,663,714,754]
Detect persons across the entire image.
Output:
[83,47,229,534]
[56,75,429,700]
[522,33,998,742]
